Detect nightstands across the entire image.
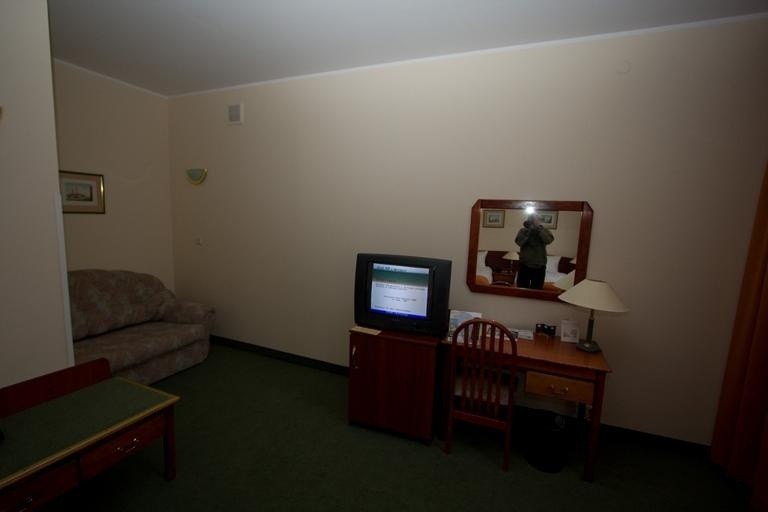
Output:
[493,268,515,285]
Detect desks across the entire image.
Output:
[0,358,181,512]
[440,328,613,480]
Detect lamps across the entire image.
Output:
[502,250,519,273]
[186,167,207,185]
[557,278,631,354]
[553,269,576,290]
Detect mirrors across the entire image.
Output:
[466,199,594,303]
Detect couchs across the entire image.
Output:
[68,269,215,386]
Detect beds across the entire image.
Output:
[475,251,498,287]
[544,256,577,292]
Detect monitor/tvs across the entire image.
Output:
[353,253,452,340]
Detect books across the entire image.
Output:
[508,328,535,340]
[449,310,482,345]
[485,322,519,341]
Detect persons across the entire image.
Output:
[514,213,555,289]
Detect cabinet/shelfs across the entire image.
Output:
[345,326,441,447]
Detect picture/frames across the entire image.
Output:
[482,210,505,228]
[560,320,580,343]
[537,211,558,229]
[59,170,105,214]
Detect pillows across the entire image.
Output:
[546,256,563,272]
[477,251,488,266]
[544,269,566,283]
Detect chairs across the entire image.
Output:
[446,319,519,472]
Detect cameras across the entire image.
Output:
[523,218,534,228]
